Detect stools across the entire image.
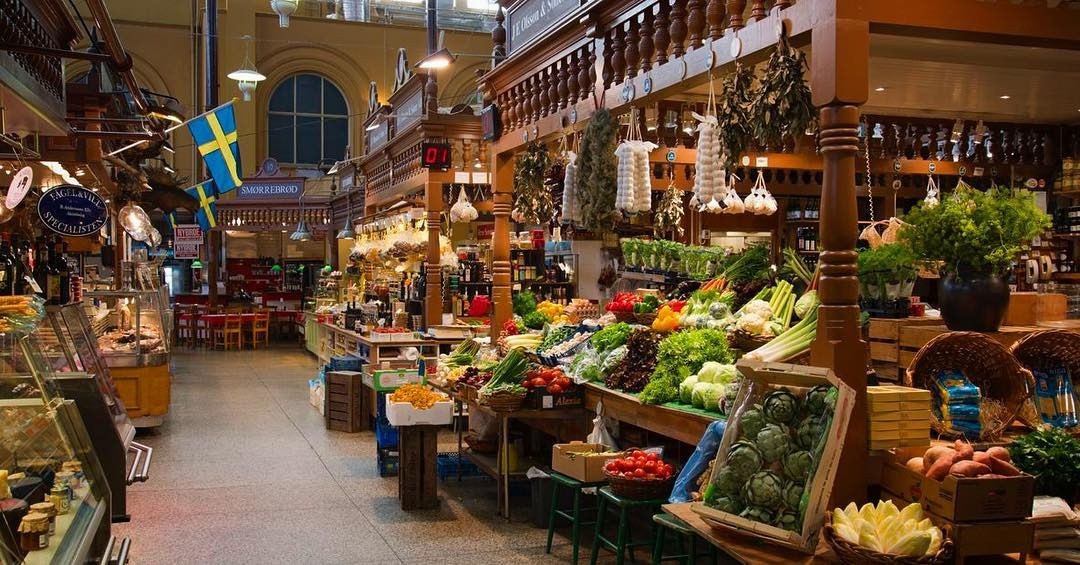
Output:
[652,514,721,565]
[546,474,636,565]
[590,486,672,565]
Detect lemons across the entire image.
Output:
[537,301,570,323]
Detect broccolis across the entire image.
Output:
[734,299,784,336]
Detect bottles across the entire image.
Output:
[501,434,519,472]
[786,197,818,273]
[1053,156,1080,274]
[17,461,87,551]
[259,256,273,266]
[0,231,69,305]
[389,229,567,317]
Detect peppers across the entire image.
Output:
[539,327,570,352]
[544,163,576,235]
[606,292,689,332]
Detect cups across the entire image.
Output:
[911,303,925,317]
[912,296,920,304]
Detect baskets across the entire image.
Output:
[907,330,1035,441]
[601,448,675,499]
[329,356,359,370]
[1011,327,1080,426]
[538,322,602,368]
[375,391,485,479]
[630,312,658,324]
[605,309,634,323]
[487,390,520,412]
[736,329,776,351]
[822,522,953,565]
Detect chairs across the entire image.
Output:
[174,302,305,353]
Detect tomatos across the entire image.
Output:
[606,449,671,479]
[523,369,571,394]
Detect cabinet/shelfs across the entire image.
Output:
[427,379,581,526]
[1051,188,1080,281]
[252,264,280,276]
[649,147,998,255]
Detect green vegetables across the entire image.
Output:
[515,292,552,334]
[620,238,743,278]
[681,289,737,331]
[1007,427,1080,504]
[572,320,735,406]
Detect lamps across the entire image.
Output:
[162,141,176,154]
[153,158,176,173]
[414,48,508,69]
[270,0,298,30]
[139,88,186,124]
[227,36,266,102]
[327,155,367,174]
[289,192,316,242]
[366,113,421,131]
[336,186,364,241]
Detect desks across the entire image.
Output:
[584,382,724,452]
[180,313,261,351]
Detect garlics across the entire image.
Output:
[561,124,726,221]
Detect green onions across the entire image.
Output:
[741,304,870,363]
[477,349,529,399]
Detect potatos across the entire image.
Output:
[905,438,1021,483]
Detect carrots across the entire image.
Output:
[701,278,727,292]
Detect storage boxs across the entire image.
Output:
[690,358,858,554]
[362,365,374,389]
[320,371,325,415]
[331,357,366,372]
[377,391,400,477]
[895,447,1036,524]
[386,393,454,428]
[880,492,1036,556]
[373,370,426,390]
[309,380,319,407]
[399,425,438,509]
[325,371,362,435]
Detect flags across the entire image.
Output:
[187,105,242,193]
[165,211,177,229]
[186,182,217,231]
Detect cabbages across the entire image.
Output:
[833,498,943,557]
[677,360,741,413]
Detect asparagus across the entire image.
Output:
[453,337,480,358]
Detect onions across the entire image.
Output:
[436,352,451,385]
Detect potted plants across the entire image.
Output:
[855,181,1054,332]
